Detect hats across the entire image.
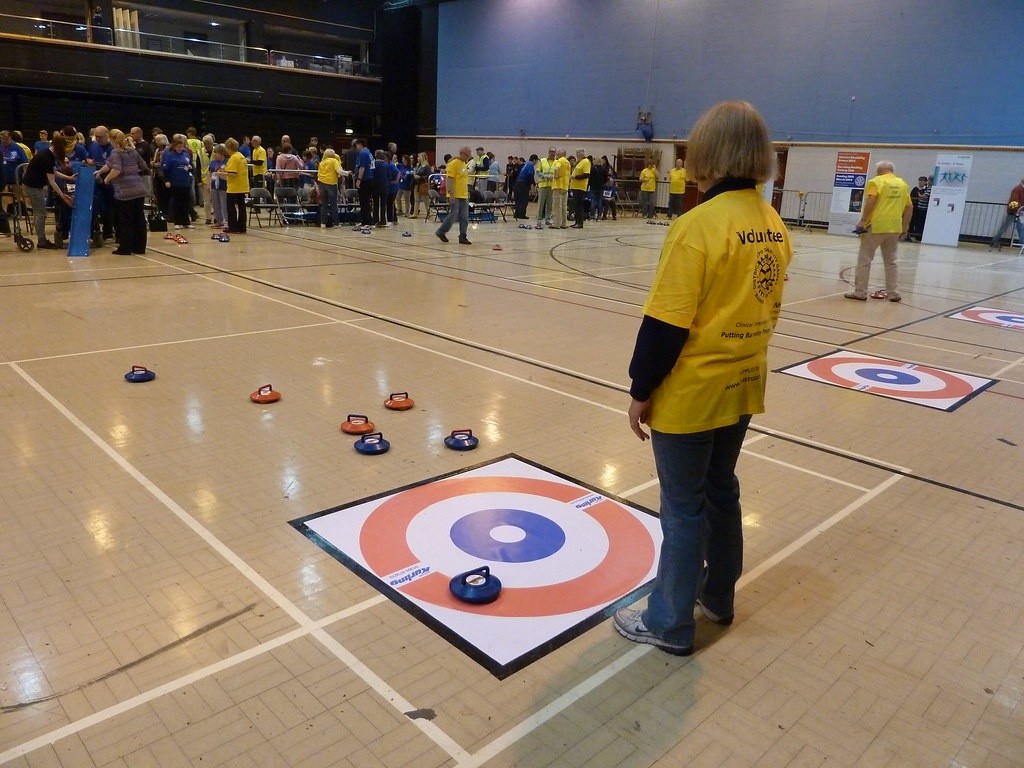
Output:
[531,154,539,160]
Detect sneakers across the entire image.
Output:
[694,597,734,625]
[612,606,694,656]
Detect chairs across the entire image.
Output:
[247,188,373,228]
[0,162,57,252]
[424,185,538,224]
[613,144,663,219]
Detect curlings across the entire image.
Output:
[164,231,188,244]
[339,414,375,435]
[383,390,414,410]
[450,566,501,604]
[869,289,887,299]
[249,384,282,404]
[646,219,670,226]
[124,365,156,383]
[211,231,231,242]
[351,224,372,235]
[518,223,542,230]
[401,231,411,237]
[492,243,503,251]
[354,431,391,455]
[784,274,789,281]
[443,428,479,452]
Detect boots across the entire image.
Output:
[53,231,64,249]
[92,231,104,248]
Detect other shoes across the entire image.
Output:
[570,223,583,228]
[112,247,145,255]
[435,229,449,242]
[844,292,867,301]
[184,225,195,229]
[459,238,472,244]
[548,225,567,229]
[222,227,247,234]
[990,241,1002,248]
[321,224,326,229]
[884,293,901,302]
[211,223,228,229]
[334,225,341,229]
[37,241,56,249]
[513,214,529,219]
[174,224,181,229]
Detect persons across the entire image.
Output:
[612,100,792,656]
[989,178,1024,253]
[906,175,933,242]
[844,161,913,301]
[0,126,690,255]
[849,191,861,212]
